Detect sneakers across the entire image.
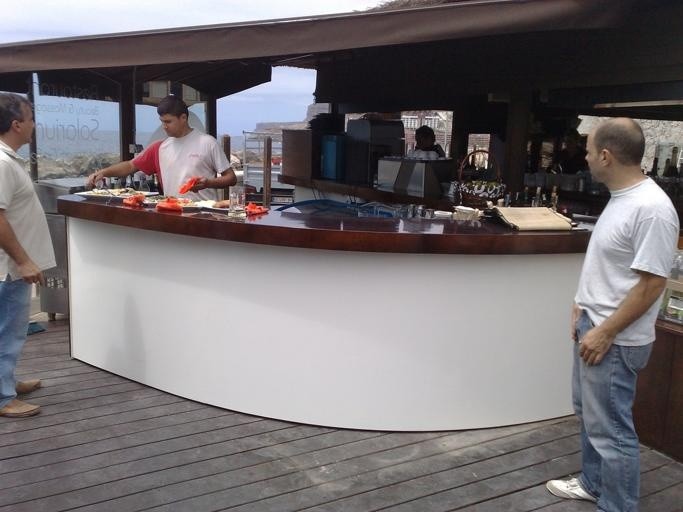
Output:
[15,379,42,394]
[546,477,598,502]
[0,398,41,418]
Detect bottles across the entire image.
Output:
[645,146,683,179]
[462,155,477,170]
[125,173,163,195]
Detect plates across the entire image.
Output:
[195,199,229,212]
[182,204,204,211]
[73,188,160,202]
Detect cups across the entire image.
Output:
[228,185,247,219]
[406,149,439,159]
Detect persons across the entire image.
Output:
[87,94,238,203]
[1,92,59,419]
[554,129,591,174]
[413,126,447,158]
[544,114,680,512]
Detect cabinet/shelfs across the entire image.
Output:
[632,318,683,464]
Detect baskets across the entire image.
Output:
[455,150,506,209]
